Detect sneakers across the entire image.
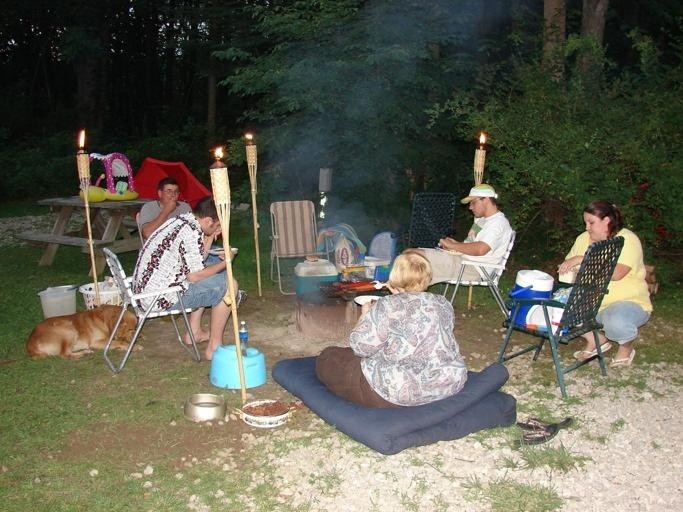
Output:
[237,289,246,308]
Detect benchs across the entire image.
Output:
[15,230,115,249]
[124,219,138,229]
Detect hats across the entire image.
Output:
[460,183,498,204]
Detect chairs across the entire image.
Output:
[103,247,200,374]
[269,200,333,295]
[497,235,625,399]
[443,231,509,320]
[391,190,457,256]
[136,212,146,247]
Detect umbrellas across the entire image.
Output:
[133,156,212,210]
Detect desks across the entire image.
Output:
[14,198,153,276]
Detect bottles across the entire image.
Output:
[238,320,248,357]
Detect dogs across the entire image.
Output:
[25,304,145,361]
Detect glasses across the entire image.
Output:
[163,190,180,195]
[469,198,480,206]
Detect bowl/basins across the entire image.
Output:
[184,393,224,422]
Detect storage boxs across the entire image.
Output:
[295,263,338,302]
[364,256,391,279]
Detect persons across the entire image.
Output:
[314,248,468,409]
[132,200,239,362]
[558,198,654,369]
[139,176,194,241]
[402,184,513,287]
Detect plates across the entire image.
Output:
[354,295,383,306]
[208,247,239,255]
[240,399,290,429]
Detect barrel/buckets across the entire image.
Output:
[37,284,79,319]
[508,270,554,324]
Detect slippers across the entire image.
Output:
[612,348,636,366]
[572,342,612,362]
[513,417,572,445]
